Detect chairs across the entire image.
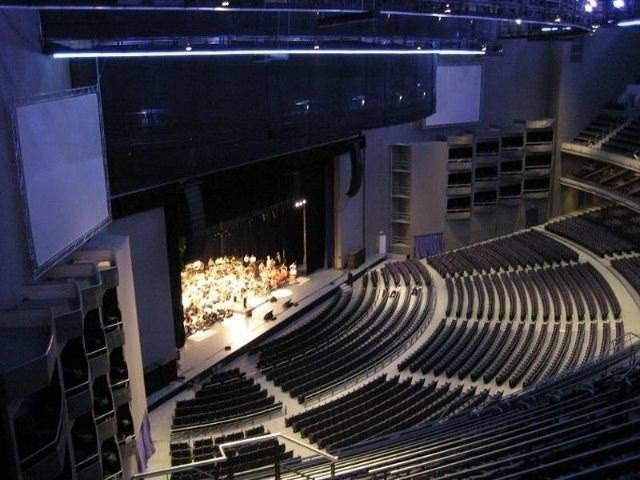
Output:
[251,287,349,367]
[560,85,640,480]
[349,215,560,368]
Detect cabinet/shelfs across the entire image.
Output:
[388,143,413,254]
